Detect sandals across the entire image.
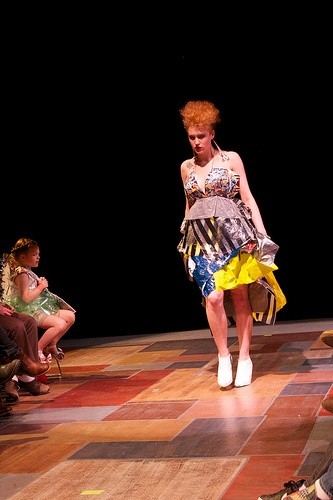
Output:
[43,345,63,362]
[36,351,51,364]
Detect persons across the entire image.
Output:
[1,301,50,395]
[177,100,288,388]
[0,324,50,403]
[1,359,20,417]
[1,238,75,363]
[257,329,333,500]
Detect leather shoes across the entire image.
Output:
[0,359,20,385]
[0,406,12,417]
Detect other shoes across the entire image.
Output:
[257,478,309,500]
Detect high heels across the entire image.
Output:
[235,358,254,387]
[4,379,19,401]
[15,352,52,377]
[217,355,234,388]
[18,378,51,395]
